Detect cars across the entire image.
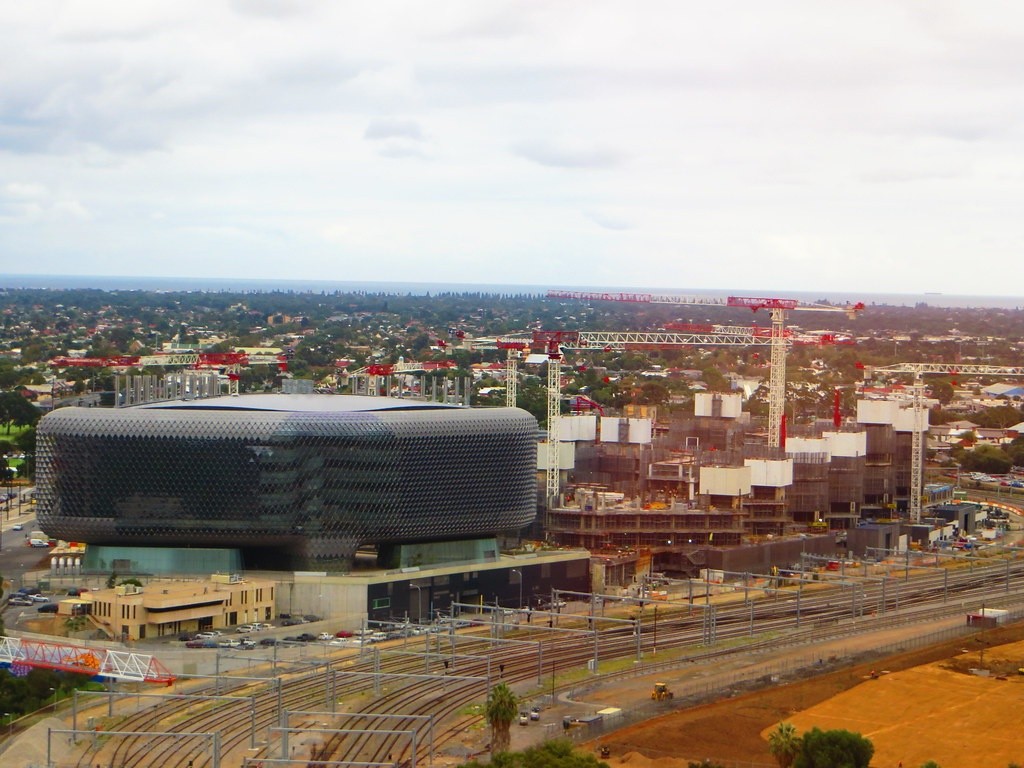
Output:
[7,583,90,615]
[183,622,439,651]
[12,523,24,531]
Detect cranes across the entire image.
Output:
[873,360,1023,523]
[504,289,867,496]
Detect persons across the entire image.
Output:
[442,659,448,675]
[499,663,506,679]
[631,615,635,626]
[640,601,644,613]
[588,615,592,629]
[547,618,553,628]
[527,612,532,624]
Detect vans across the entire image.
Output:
[30,539,48,548]
